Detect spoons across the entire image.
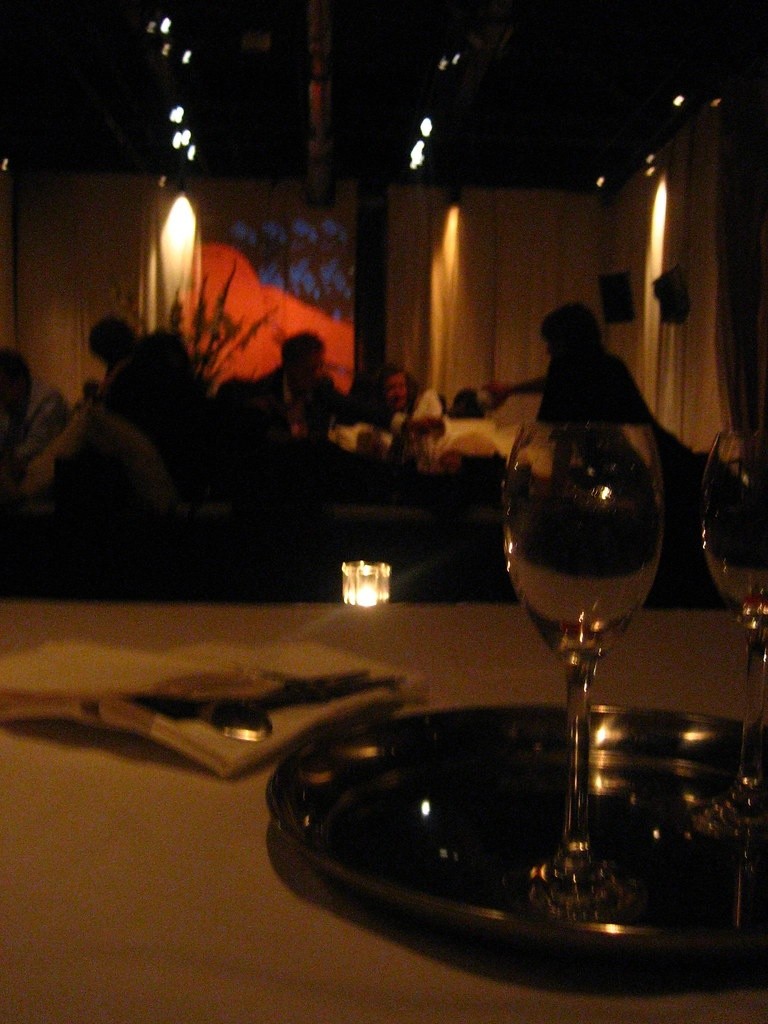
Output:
[81,693,272,743]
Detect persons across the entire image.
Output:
[0,301,698,521]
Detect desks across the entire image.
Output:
[0,598,768,1024]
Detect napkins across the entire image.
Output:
[1,636,418,777]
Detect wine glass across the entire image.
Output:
[500,423,665,926]
[681,433,768,847]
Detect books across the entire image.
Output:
[0,637,410,782]
